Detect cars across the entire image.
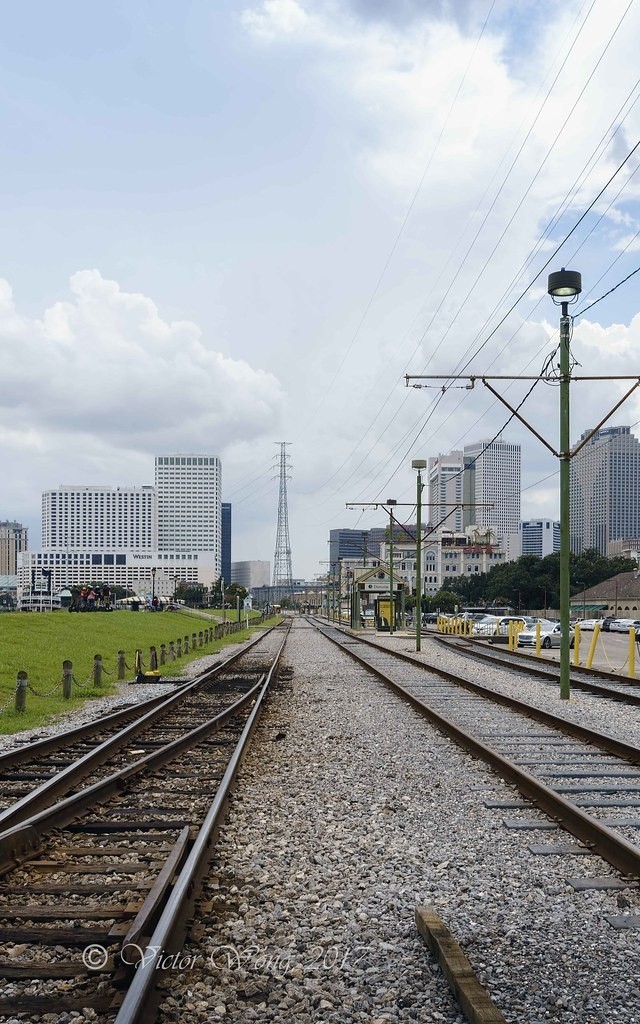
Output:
[522,616,537,621]
[394,612,411,622]
[599,619,605,629]
[618,619,640,634]
[609,618,626,633]
[419,612,425,619]
[422,612,449,625]
[533,618,553,624]
[577,619,601,631]
[446,613,455,618]
[518,623,583,650]
[359,609,376,617]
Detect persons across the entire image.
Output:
[141,595,159,613]
[69,583,80,607]
[87,586,99,607]
[86,585,91,600]
[79,586,87,608]
[103,583,111,607]
[94,585,102,607]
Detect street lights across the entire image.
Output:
[151,568,157,611]
[315,554,347,629]
[358,528,372,630]
[511,577,627,620]
[173,576,177,607]
[381,498,402,636]
[403,266,639,701]
[344,459,496,653]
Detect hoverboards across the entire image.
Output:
[64,594,114,613]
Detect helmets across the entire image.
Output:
[96,586,99,588]
[82,587,87,590]
[90,586,94,588]
[103,583,108,585]
[87,585,91,588]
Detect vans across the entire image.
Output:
[472,616,527,638]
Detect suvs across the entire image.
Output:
[454,612,474,626]
[603,615,625,631]
[465,613,491,630]
[566,617,584,626]
